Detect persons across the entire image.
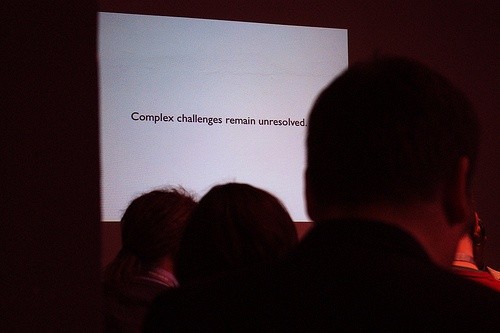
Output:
[233,55,500,333]
[457,210,500,289]
[103,190,202,333]
[141,181,302,333]
